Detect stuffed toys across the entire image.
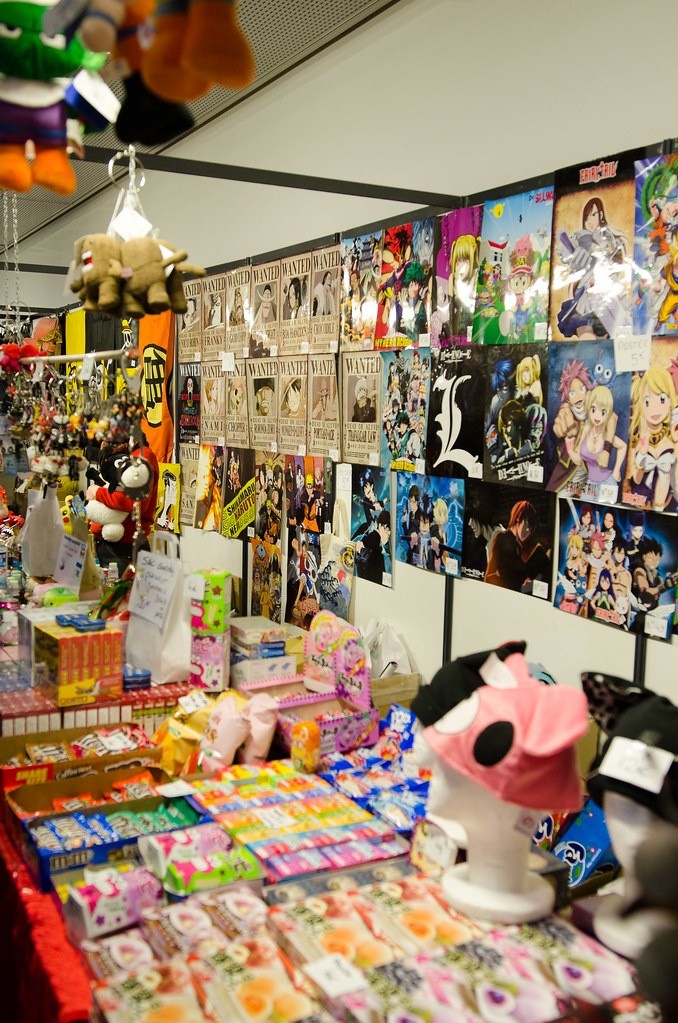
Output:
[0,0,253,195]
[70,234,207,317]
[0,574,81,645]
[61,442,144,542]
[0,337,56,379]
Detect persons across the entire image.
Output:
[85,426,159,578]
[423,654,591,893]
[409,641,528,771]
[585,695,678,919]
[0,469,29,527]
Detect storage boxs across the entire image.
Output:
[0,723,211,894]
[238,608,421,754]
[188,567,231,693]
[229,650,296,690]
[17,606,123,708]
